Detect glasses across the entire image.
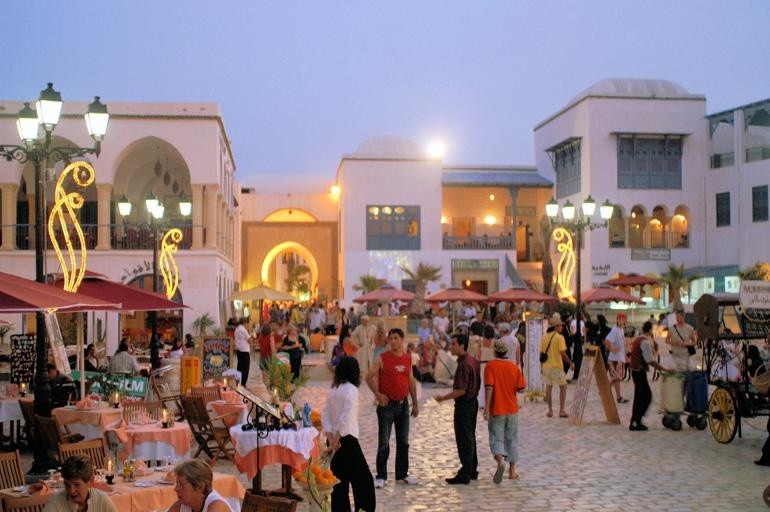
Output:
[63,458,85,469]
[175,481,191,489]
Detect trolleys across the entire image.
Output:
[652,365,716,432]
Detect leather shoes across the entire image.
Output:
[446,476,470,484]
[755,459,770,466]
[456,472,478,480]
[629,423,648,430]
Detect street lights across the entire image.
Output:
[0,81,110,477]
[545,193,616,387]
[117,188,194,380]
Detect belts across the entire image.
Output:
[391,401,408,404]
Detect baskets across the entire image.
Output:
[752,363,770,395]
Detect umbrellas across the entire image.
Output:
[0,267,192,401]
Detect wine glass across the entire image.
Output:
[46,470,58,487]
[95,469,104,481]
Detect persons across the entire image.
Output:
[267,301,696,488]
[754,420,769,467]
[49,316,377,512]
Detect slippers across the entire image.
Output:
[493,463,506,484]
[560,414,569,417]
[547,413,553,417]
[509,474,520,481]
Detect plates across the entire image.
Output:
[74,407,94,411]
[134,481,154,487]
[156,478,172,485]
[13,486,27,492]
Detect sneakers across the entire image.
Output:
[374,478,388,489]
[395,477,417,485]
[617,397,630,402]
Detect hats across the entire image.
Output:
[493,339,508,353]
[498,322,511,330]
[546,318,566,329]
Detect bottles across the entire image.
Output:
[109,396,112,407]
[241,408,266,431]
[123,459,136,482]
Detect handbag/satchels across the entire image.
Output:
[566,362,575,381]
[688,346,696,355]
[539,352,547,362]
